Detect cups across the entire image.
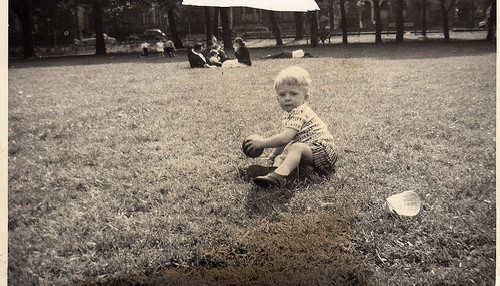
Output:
[386,191,420,216]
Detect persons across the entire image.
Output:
[479,22,486,31]
[247,66,339,189]
[260,48,313,59]
[141,38,178,57]
[188,35,252,67]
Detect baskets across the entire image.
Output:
[383,188,421,223]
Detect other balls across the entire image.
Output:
[243,140,264,158]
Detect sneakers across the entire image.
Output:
[253,172,287,188]
[247,165,278,178]
